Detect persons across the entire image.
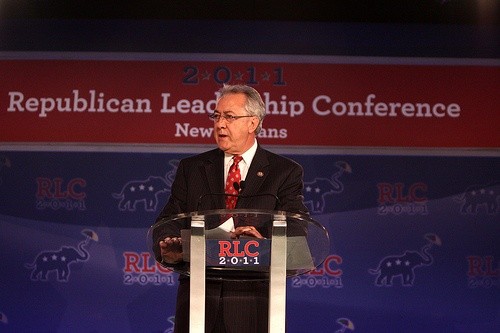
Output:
[152,83,313,332]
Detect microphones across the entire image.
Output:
[196,180,246,211]
[233,182,280,212]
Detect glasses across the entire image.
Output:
[209,113,256,122]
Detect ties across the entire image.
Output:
[225,154,242,218]
